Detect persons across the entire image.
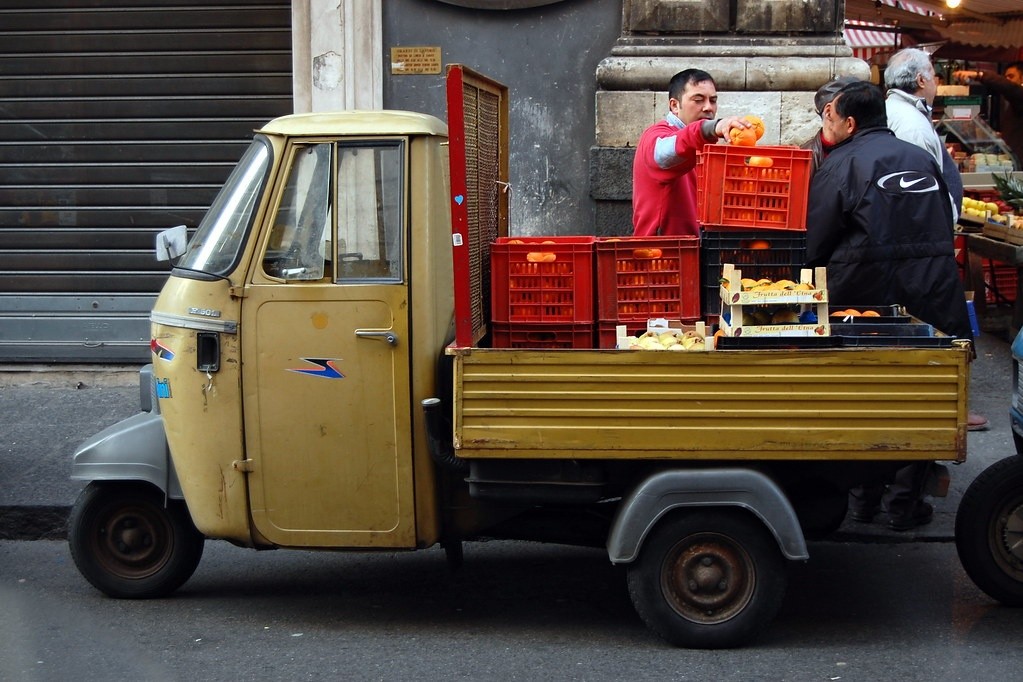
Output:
[633,70,751,240]
[799,78,858,181]
[883,48,988,431]
[953,63,1023,171]
[791,82,976,530]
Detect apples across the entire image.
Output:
[991,213,1023,231]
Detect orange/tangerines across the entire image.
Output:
[504,239,572,317]
[721,115,788,223]
[829,308,880,317]
[614,249,681,314]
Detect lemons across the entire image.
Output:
[960,197,998,218]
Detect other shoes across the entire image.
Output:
[849,506,879,521]
[966,414,987,431]
[886,503,933,530]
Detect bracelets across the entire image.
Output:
[975,70,979,81]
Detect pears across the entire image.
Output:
[627,330,706,351]
[722,278,814,325]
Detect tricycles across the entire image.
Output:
[952,324,1023,609]
[66,113,971,649]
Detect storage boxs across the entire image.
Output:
[490,324,595,348]
[697,141,818,352]
[596,236,700,324]
[982,265,1019,301]
[488,236,595,324]
[598,323,647,349]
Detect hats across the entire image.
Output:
[814,76,862,118]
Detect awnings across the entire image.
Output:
[841,0,945,61]
